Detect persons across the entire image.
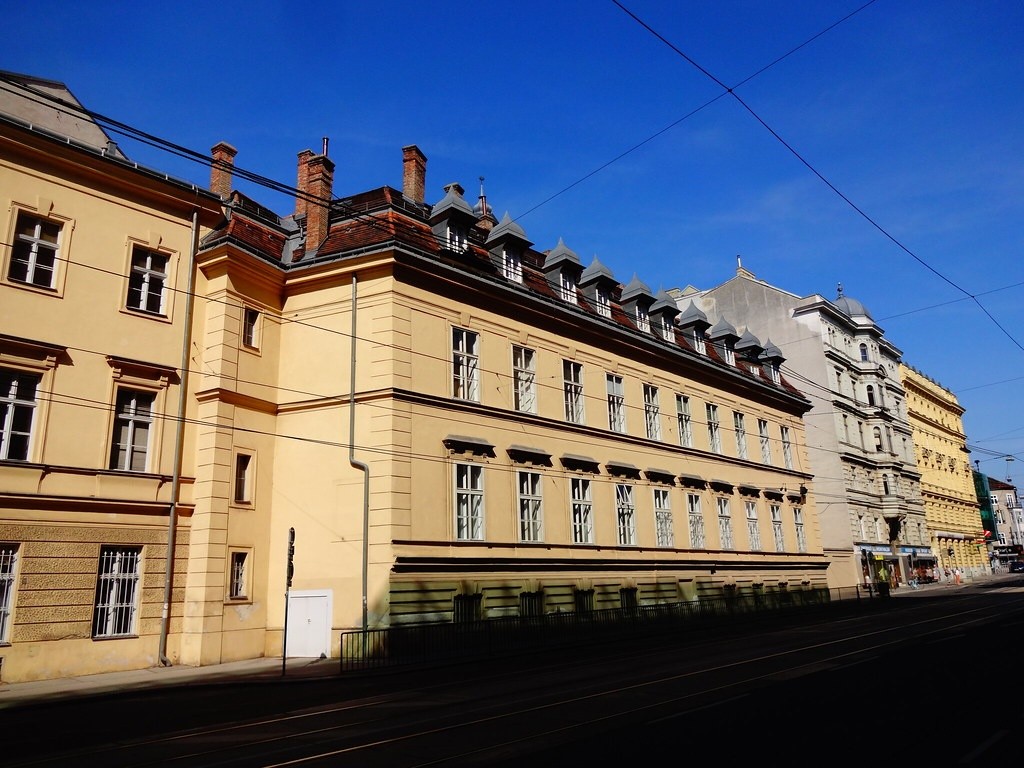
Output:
[909,571,918,589]
[863,565,872,588]
[926,564,961,586]
